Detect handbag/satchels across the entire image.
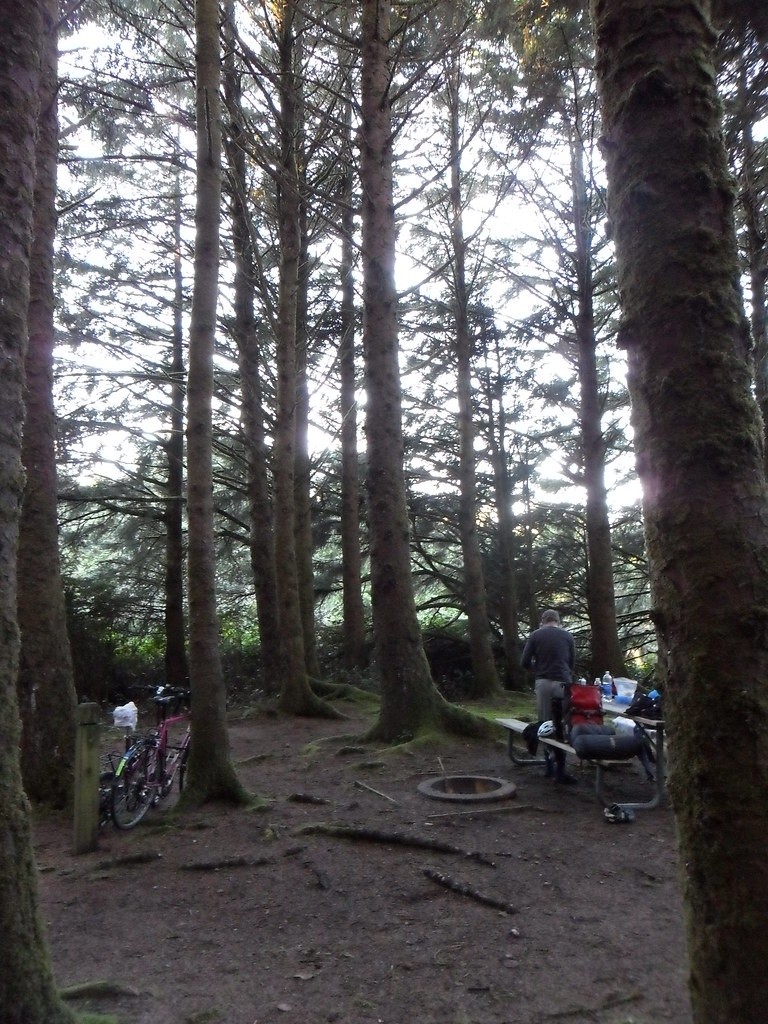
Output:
[567,683,603,727]
[626,691,664,720]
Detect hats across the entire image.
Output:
[541,609,559,621]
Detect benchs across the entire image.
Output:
[495,717,669,810]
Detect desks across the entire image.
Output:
[602,699,667,810]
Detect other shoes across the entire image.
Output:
[544,764,558,775]
[556,772,577,784]
[604,804,635,823]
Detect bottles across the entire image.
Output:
[602,671,613,702]
[594,678,601,687]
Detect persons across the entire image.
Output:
[519,610,578,783]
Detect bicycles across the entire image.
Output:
[99,674,193,829]
